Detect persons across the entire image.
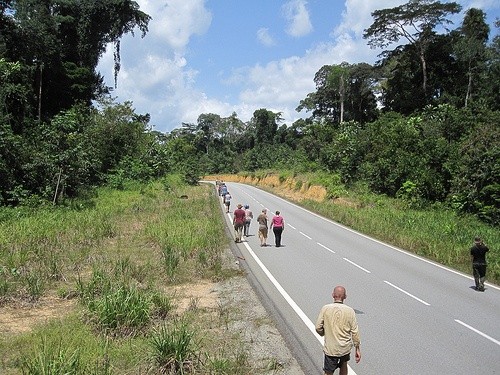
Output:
[270,211,284,247]
[470,237,489,291]
[232,204,246,243]
[242,204,253,237]
[218,179,231,214]
[316,286,361,375]
[257,209,270,247]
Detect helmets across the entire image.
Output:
[244,204,250,209]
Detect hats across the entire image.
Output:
[473,236,483,244]
[237,203,243,208]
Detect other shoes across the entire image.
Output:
[261,242,267,247]
